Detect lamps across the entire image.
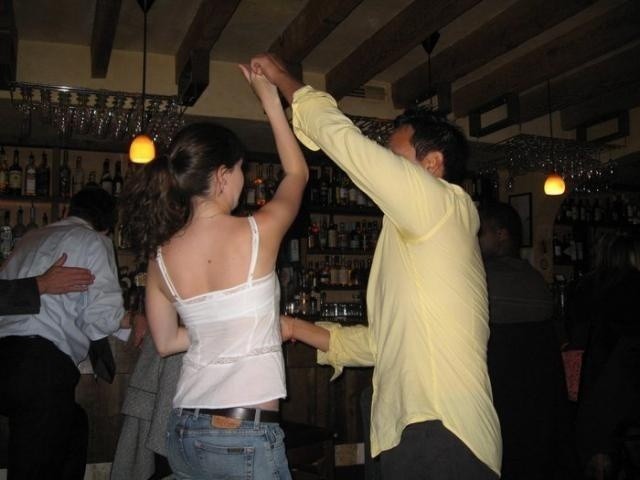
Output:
[544,48,567,196]
[129,1,156,165]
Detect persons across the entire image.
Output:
[1,182,146,461]
[1,252,95,316]
[477,198,555,323]
[145,61,308,479]
[250,53,503,477]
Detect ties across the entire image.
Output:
[89,338,116,384]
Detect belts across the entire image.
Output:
[183,408,279,422]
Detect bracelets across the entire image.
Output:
[128,309,139,330]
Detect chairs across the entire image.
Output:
[486,318,558,479]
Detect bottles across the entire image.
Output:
[460,165,499,201]
[552,187,640,307]
[238,159,383,324]
[0,139,148,314]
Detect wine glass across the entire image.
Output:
[53,102,184,151]
[505,139,618,193]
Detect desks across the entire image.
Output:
[337,316,373,469]
[76,314,334,464]
